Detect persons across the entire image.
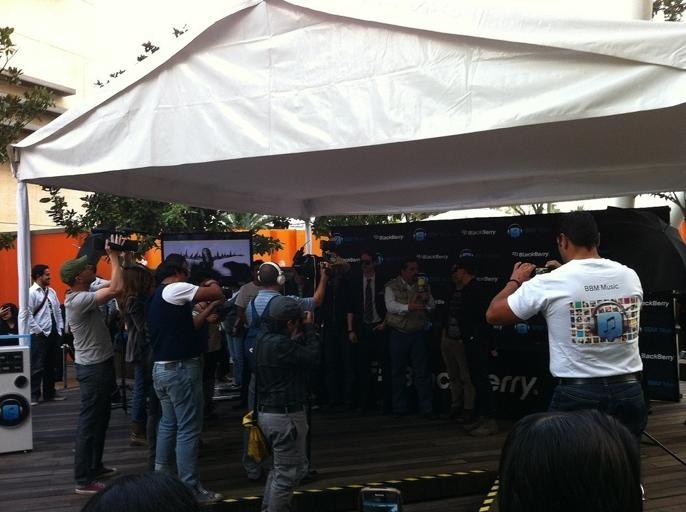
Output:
[0,303,19,357]
[498,408,646,512]
[60,233,496,510]
[486,211,648,441]
[28,263,65,406]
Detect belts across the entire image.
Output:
[560,374,641,385]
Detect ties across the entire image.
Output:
[365,278,375,322]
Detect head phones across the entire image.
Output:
[257,262,286,287]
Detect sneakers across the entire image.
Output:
[93,466,118,480]
[308,395,501,439]
[74,481,107,495]
[189,487,226,503]
[44,394,67,402]
[31,399,39,406]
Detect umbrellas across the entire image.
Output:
[599,206,686,293]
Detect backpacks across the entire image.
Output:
[243,319,264,374]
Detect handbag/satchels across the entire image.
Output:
[242,409,272,464]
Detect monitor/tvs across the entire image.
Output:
[161,232,252,280]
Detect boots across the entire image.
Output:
[128,422,146,446]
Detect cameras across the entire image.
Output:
[532,266,550,280]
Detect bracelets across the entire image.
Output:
[509,279,521,288]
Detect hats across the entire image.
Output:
[59,254,88,284]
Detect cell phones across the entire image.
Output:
[357,488,402,512]
